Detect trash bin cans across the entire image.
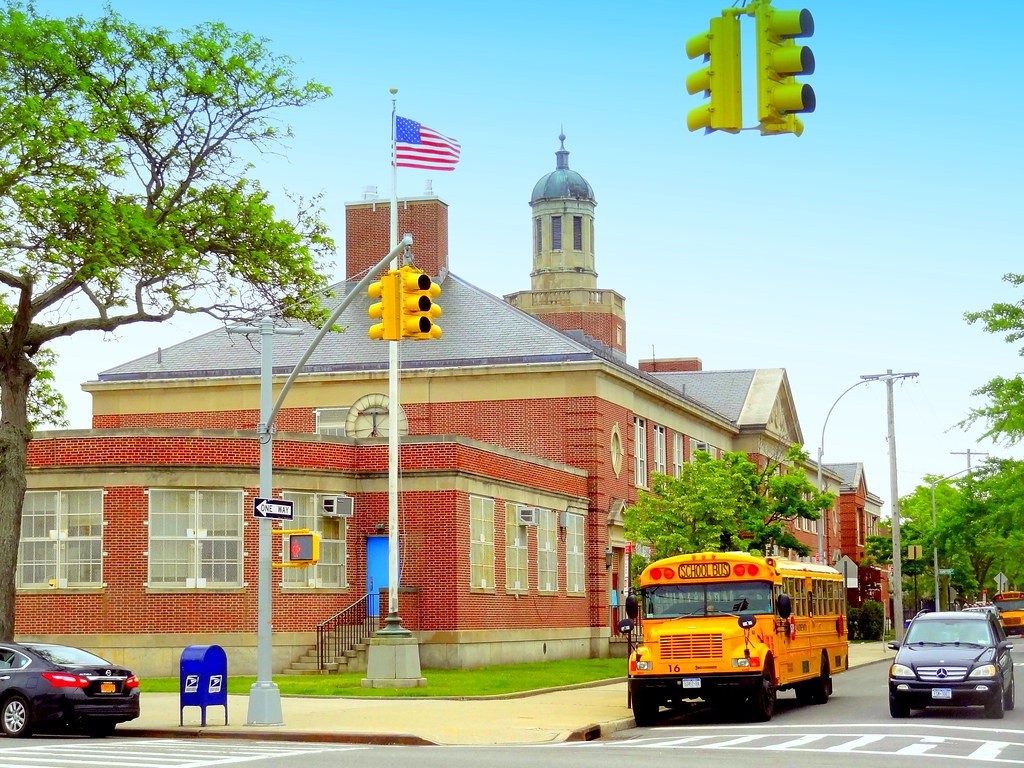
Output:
[180,643,230,728]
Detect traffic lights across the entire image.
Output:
[756,5,817,134]
[400,267,432,339]
[289,533,321,563]
[684,13,743,134]
[368,271,398,341]
[428,281,444,339]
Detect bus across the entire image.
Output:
[616,550,850,730]
[992,590,1024,637]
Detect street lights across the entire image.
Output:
[816,372,907,567]
[931,464,987,612]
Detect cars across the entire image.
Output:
[0,640,140,738]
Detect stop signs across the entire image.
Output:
[838,616,844,638]
[790,617,796,641]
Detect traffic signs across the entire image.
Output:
[254,494,295,521]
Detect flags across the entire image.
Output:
[391,111,461,171]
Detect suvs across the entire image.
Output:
[960,602,1004,630]
[885,608,1015,719]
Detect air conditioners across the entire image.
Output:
[697,442,710,455]
[518,507,541,527]
[322,496,354,518]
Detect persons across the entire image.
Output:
[733,595,751,610]
[954,594,962,611]
